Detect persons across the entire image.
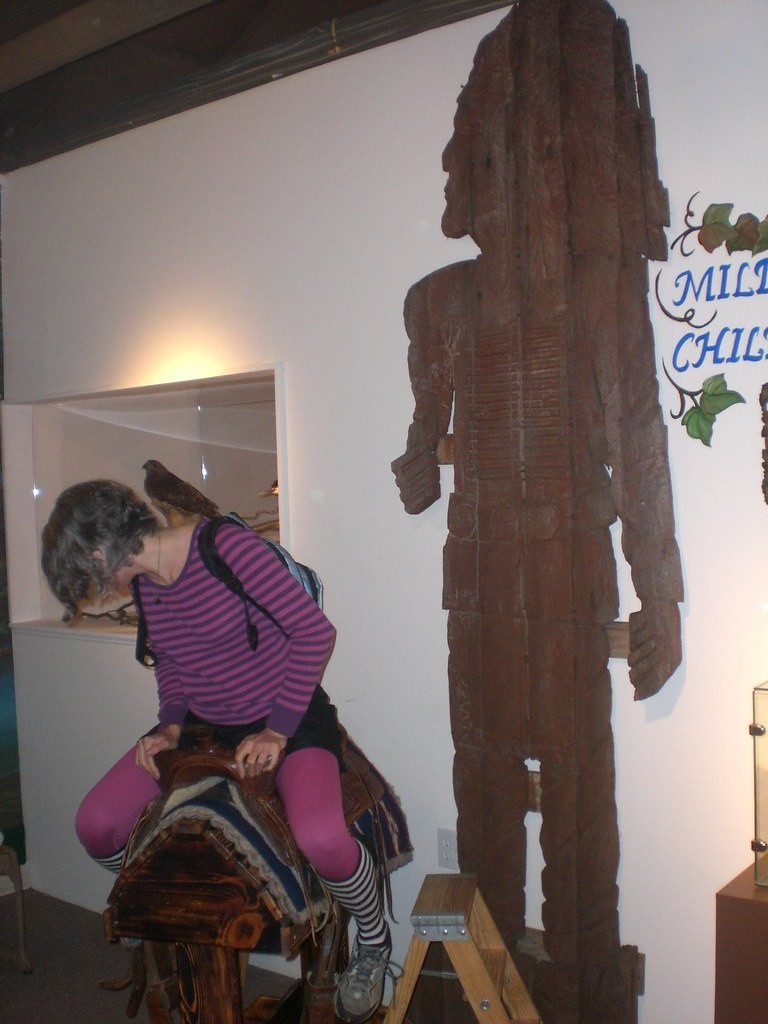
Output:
[41,481,405,1024]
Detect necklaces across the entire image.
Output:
[144,527,164,606]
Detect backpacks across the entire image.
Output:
[133,511,323,668]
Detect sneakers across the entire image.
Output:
[332,922,404,1024]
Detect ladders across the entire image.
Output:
[382,873,545,1024]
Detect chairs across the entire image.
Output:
[0,831,34,974]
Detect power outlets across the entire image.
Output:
[437,828,459,869]
